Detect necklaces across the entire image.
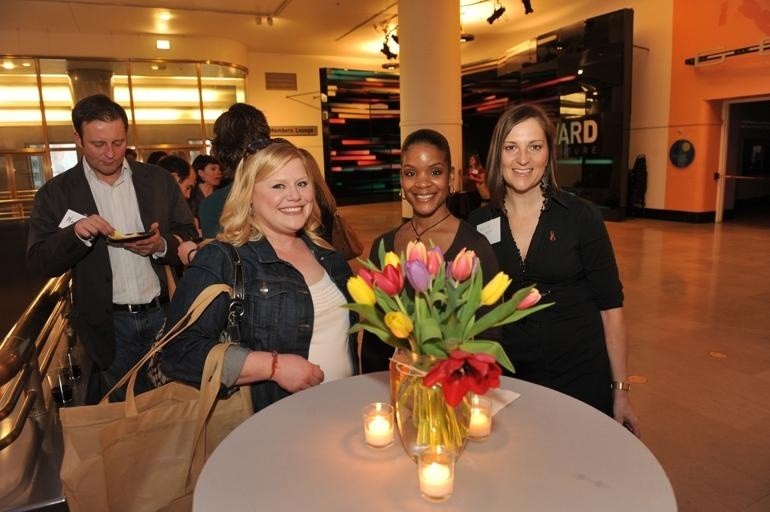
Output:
[411,214,451,244]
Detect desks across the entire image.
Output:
[191,370,677,512]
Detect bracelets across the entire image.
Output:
[270,351,277,378]
[611,382,632,392]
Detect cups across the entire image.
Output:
[47,366,73,405]
[417,447,456,505]
[362,401,396,447]
[54,345,82,384]
[465,394,492,440]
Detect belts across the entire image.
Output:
[113,296,169,313]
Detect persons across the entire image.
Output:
[359,129,502,374]
[24,93,199,401]
[469,105,639,439]
[159,138,359,412]
[127,104,270,302]
[465,151,486,191]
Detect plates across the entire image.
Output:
[104,233,154,243]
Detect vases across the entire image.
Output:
[388,345,471,463]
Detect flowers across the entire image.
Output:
[340,237,555,451]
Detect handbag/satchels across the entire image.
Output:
[146,323,240,400]
[59,380,254,512]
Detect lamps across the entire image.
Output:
[380,0,533,59]
